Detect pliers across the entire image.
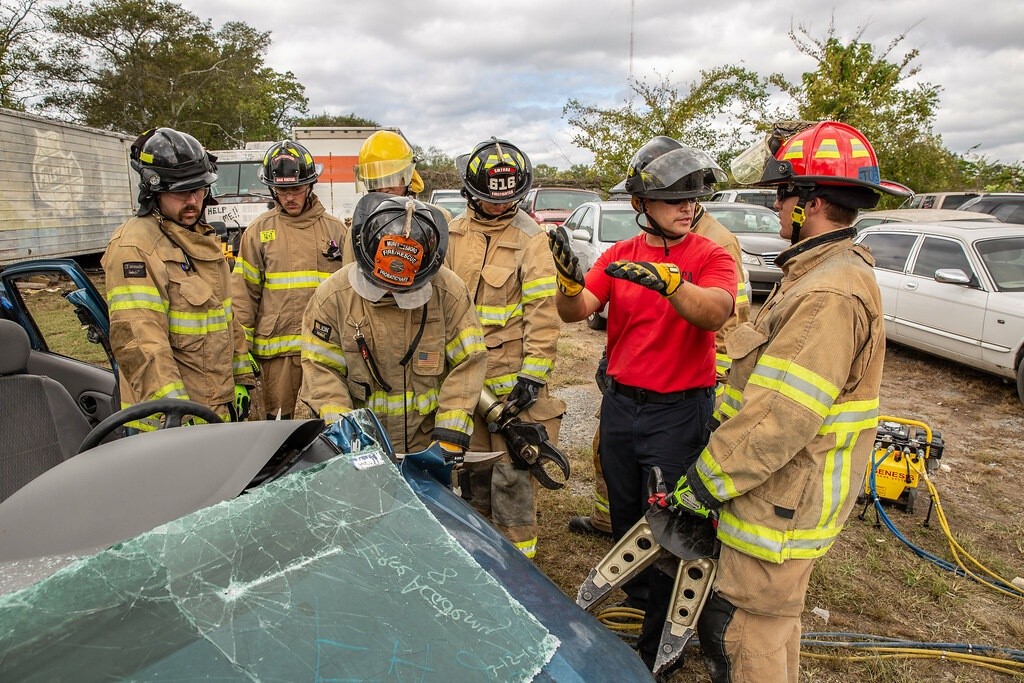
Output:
[576,465,722,676]
[475,386,570,490]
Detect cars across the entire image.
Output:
[698,200,792,295]
[427,188,469,218]
[851,221,1024,405]
[0,255,657,683]
[849,208,1024,267]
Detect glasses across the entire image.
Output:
[169,188,209,201]
[776,184,814,201]
[665,198,697,204]
[270,184,309,196]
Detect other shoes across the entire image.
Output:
[602,601,635,623]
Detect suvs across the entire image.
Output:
[706,187,782,230]
[955,192,1024,225]
[897,190,985,210]
[516,186,618,238]
[557,178,753,331]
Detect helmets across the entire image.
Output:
[456,136,534,203]
[625,136,714,200]
[130,127,219,193]
[358,132,414,179]
[258,140,322,186]
[750,121,914,196]
[351,192,449,294]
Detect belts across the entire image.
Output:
[611,376,712,403]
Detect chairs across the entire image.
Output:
[570,199,585,210]
[988,249,1021,260]
[736,219,747,230]
[217,173,227,187]
[603,218,616,233]
[0,318,99,503]
[629,218,645,232]
[719,217,730,228]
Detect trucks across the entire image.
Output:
[202,122,418,254]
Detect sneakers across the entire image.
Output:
[569,516,613,537]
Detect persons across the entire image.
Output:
[341,131,452,266]
[571,202,756,537]
[100,127,261,434]
[550,135,741,676]
[291,193,488,502]
[441,136,561,561]
[232,140,348,418]
[656,121,915,682]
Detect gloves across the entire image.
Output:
[669,473,714,529]
[231,384,252,422]
[180,417,195,426]
[604,259,682,298]
[440,446,463,470]
[507,381,538,411]
[548,227,585,296]
